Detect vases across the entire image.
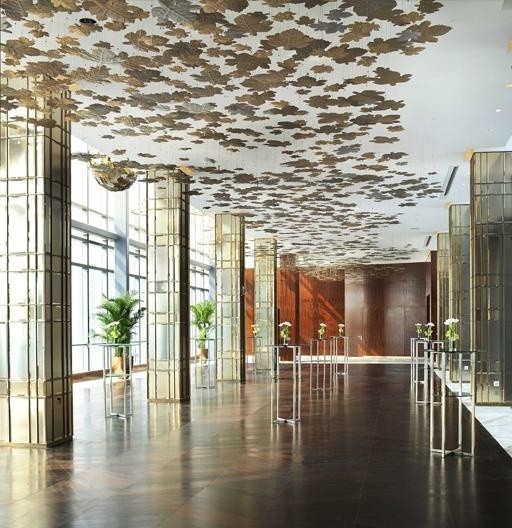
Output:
[416,332,421,339]
[338,331,343,338]
[318,333,324,340]
[448,337,456,352]
[253,332,257,337]
[281,334,288,346]
[427,335,432,343]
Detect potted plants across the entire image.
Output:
[190,300,215,362]
[90,290,148,379]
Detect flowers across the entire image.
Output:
[317,322,327,338]
[423,322,435,338]
[336,323,344,335]
[444,318,460,346]
[250,324,259,334]
[414,322,422,335]
[278,322,292,344]
